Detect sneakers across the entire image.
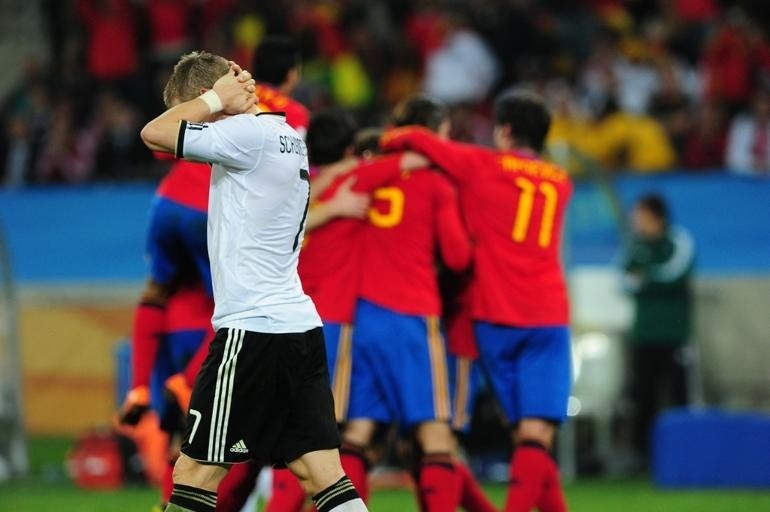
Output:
[163,373,193,416]
[120,387,150,426]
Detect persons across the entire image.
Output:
[117,34,574,511]
[606,193,696,477]
[1,1,769,190]
